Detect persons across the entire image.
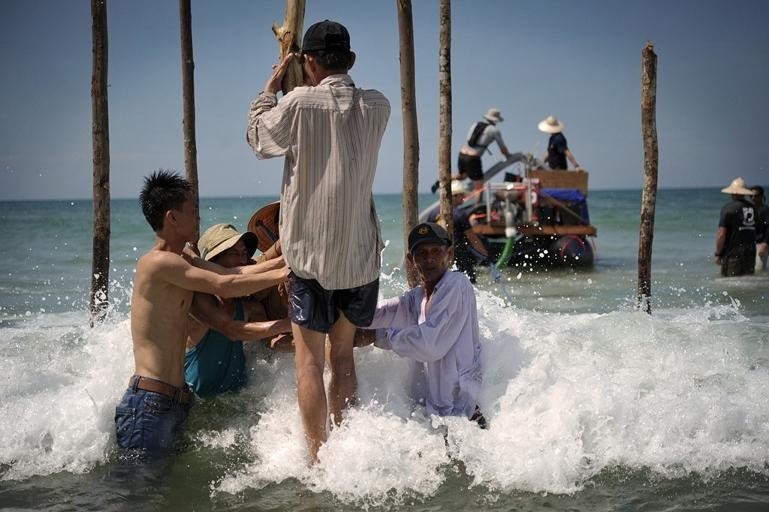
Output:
[248,200,296,351]
[746,185,768,270]
[537,115,584,174]
[349,222,488,430]
[105,167,299,476]
[430,108,513,210]
[713,176,759,278]
[185,222,293,401]
[246,19,391,477]
[427,179,489,285]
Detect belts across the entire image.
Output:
[128,375,196,404]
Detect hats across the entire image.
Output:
[537,116,565,133]
[197,222,258,263]
[721,176,755,196]
[246,201,280,253]
[408,222,453,256]
[300,19,351,65]
[482,109,504,122]
